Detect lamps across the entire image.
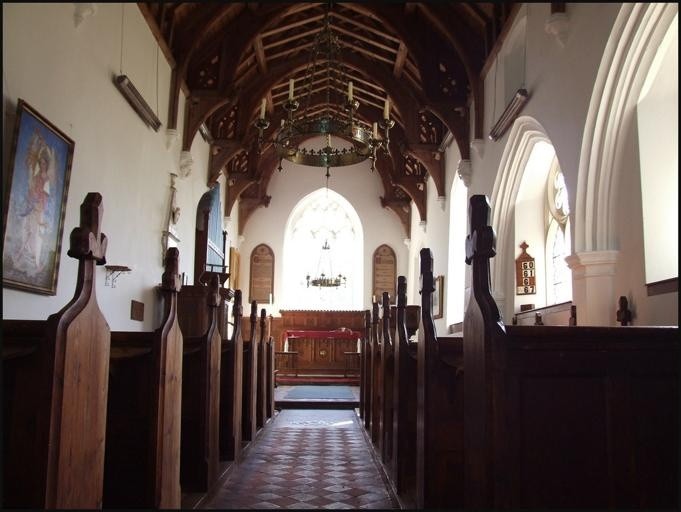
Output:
[306,160,347,290]
[487,5,528,143]
[256,3,396,178]
[117,4,162,132]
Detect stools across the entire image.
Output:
[344,351,361,379]
[274,349,299,379]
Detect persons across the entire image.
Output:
[11,155,51,279]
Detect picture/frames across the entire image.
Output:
[431,277,443,319]
[0,97,77,296]
